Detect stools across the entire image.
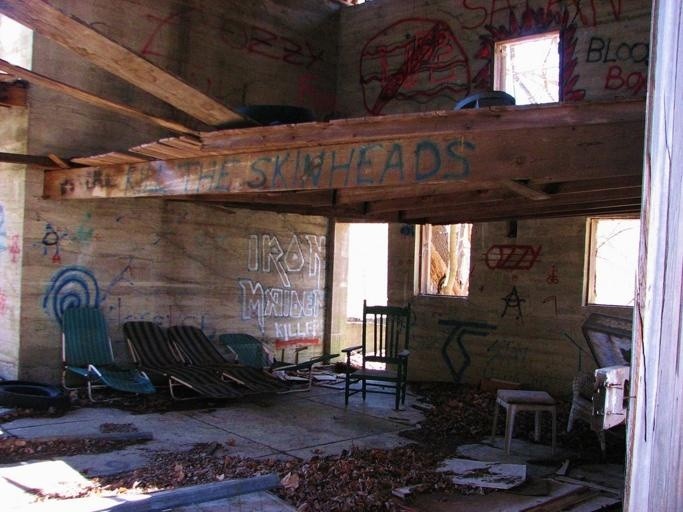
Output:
[491,389,556,456]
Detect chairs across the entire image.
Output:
[568,369,606,453]
[168,323,280,397]
[217,331,340,393]
[454,91,515,107]
[342,299,410,409]
[122,320,239,410]
[60,308,118,407]
